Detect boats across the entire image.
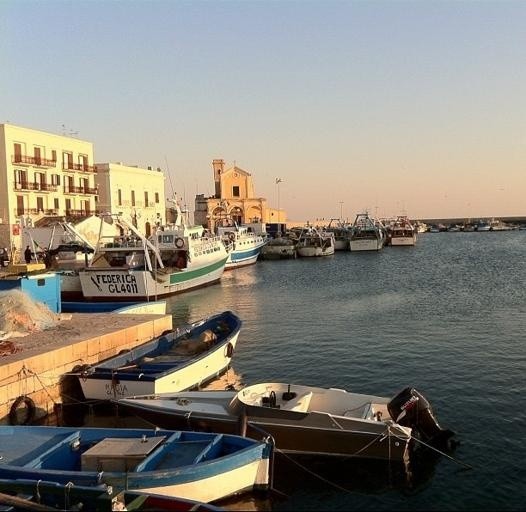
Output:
[78,309,244,404]
[78,205,233,302]
[0,478,237,512]
[264,218,349,259]
[413,219,524,233]
[0,424,277,506]
[388,215,417,246]
[17,214,120,300]
[112,381,448,481]
[215,218,270,269]
[348,211,387,251]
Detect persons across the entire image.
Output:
[24,246,31,263]
